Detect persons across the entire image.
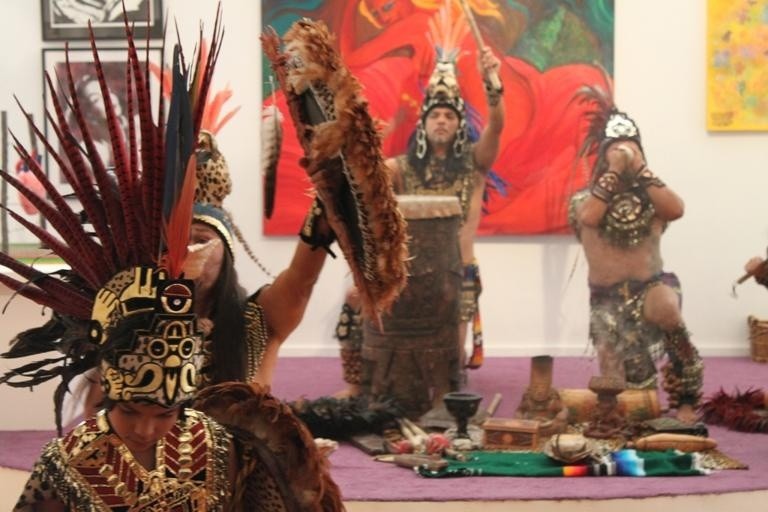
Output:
[84,148,340,422]
[1,342,296,512]
[335,45,504,431]
[745,257,768,291]
[58,72,144,180]
[567,111,704,425]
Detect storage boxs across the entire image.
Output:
[484,417,540,451]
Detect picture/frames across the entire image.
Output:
[37,43,167,253]
[38,0,165,43]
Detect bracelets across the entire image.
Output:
[298,193,339,259]
[590,169,622,202]
[484,84,500,107]
[634,163,666,191]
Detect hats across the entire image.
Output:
[420,57,466,120]
[189,203,235,264]
[546,433,591,464]
[598,112,642,168]
[88,264,206,411]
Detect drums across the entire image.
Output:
[556,390,660,427]
[360,195,463,420]
